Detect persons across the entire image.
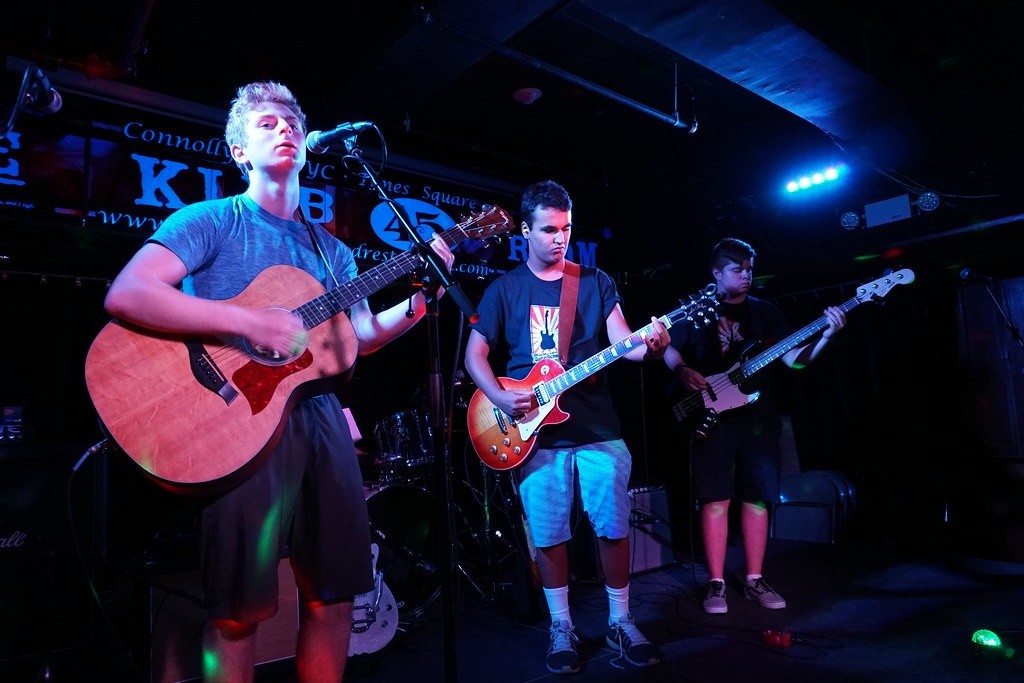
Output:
[464,181,671,674]
[105,80,454,683]
[664,237,847,614]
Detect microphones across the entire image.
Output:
[24,65,63,115]
[961,269,996,282]
[304,120,373,154]
[640,518,660,525]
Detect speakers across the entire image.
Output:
[0,440,98,672]
[567,481,677,583]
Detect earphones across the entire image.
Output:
[523,228,528,233]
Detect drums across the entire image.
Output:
[372,408,438,475]
[361,482,459,623]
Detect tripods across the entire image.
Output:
[458,459,529,590]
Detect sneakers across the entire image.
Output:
[703,580,728,613]
[546,620,583,673]
[744,577,786,609]
[606,613,660,669]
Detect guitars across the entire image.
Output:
[82,200,515,506]
[648,264,918,459]
[464,281,723,476]
